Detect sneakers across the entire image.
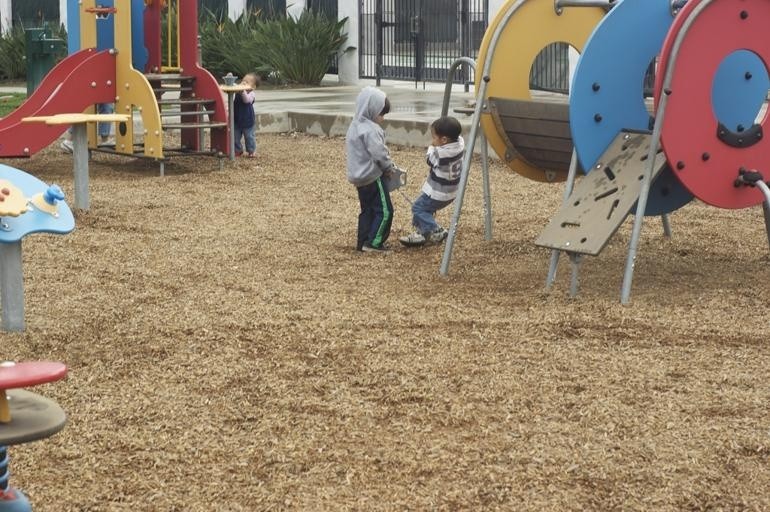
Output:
[358,241,390,253]
[430,228,448,242]
[236,151,243,157]
[249,152,256,157]
[398,231,426,245]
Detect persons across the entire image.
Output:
[346,87,395,253]
[234,72,260,157]
[399,117,465,245]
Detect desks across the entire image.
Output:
[20,112,132,210]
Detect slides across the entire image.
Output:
[0,48,117,158]
